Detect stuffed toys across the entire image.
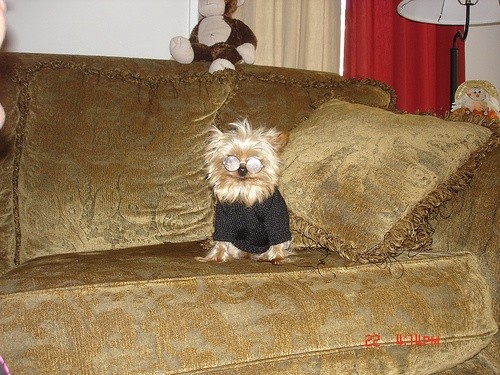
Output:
[170,0,258,73]
[447,79,500,120]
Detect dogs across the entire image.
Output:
[196,117,293,263]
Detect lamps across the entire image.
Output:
[397,0,500,114]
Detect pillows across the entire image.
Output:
[274,92,500,264]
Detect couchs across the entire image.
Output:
[0,51,500,375]
